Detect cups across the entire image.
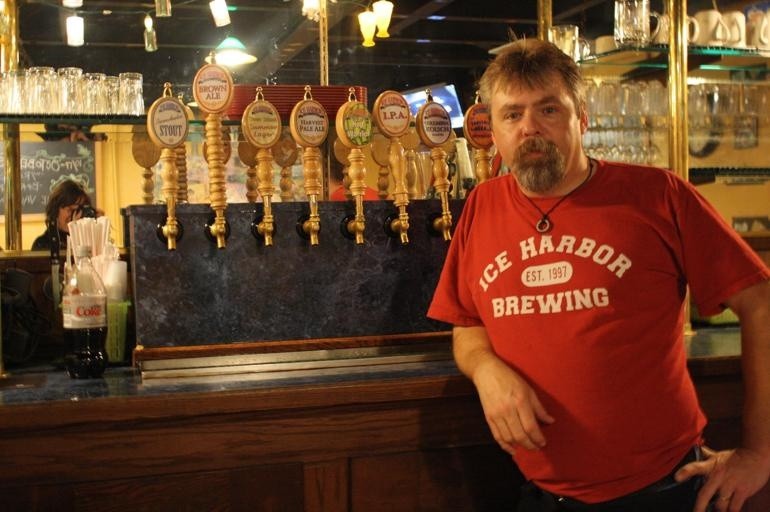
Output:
[686,10,770,50]
[613,0,662,50]
[0,67,146,116]
[548,24,590,67]
[594,35,617,55]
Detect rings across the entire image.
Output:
[718,494,732,503]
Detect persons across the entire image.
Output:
[23,178,108,250]
[420,36,770,512]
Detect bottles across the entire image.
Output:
[62,245,110,379]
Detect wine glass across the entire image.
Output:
[583,77,669,165]
[687,83,770,130]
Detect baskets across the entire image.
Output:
[60,300,132,363]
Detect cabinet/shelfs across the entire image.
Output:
[0,112,145,126]
[534,0,769,331]
[0,368,770,511]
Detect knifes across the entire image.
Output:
[49,218,61,313]
